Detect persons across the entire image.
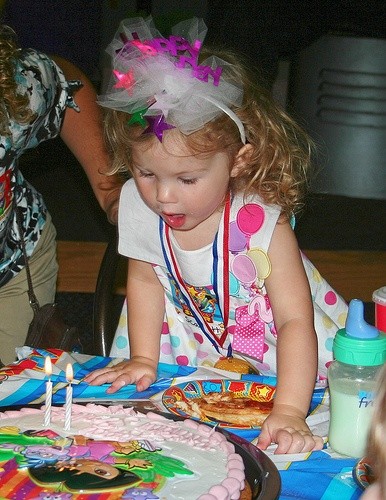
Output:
[0,23,126,369]
[81,14,349,455]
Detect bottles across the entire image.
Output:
[326,298,386,457]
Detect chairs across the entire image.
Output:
[270,33,386,200]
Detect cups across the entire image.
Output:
[371,286,386,333]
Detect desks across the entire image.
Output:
[1,347,370,500]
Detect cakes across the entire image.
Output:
[0,402,245,500]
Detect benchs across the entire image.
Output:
[57,241,386,303]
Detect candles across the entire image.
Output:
[45,356,52,426]
[65,363,74,429]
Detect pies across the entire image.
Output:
[189,391,274,425]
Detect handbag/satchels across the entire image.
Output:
[24,303,82,351]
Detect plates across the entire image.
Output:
[0,404,281,500]
[160,379,276,430]
